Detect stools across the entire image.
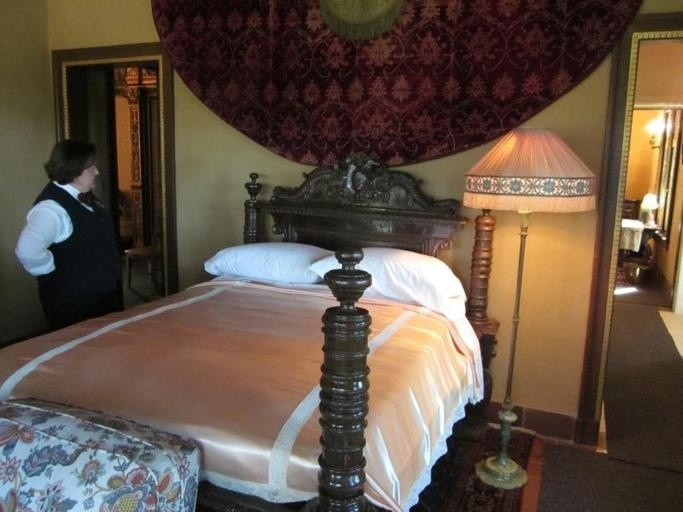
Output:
[123,245,161,291]
[0,394,204,512]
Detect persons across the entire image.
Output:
[14,137,125,332]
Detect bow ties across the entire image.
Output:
[78,191,95,206]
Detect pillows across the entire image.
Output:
[309,246,467,319]
[202,242,337,285]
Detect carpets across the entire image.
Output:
[196,417,546,512]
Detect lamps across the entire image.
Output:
[461,126,598,492]
[644,119,666,149]
[640,192,659,226]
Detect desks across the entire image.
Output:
[616,217,659,280]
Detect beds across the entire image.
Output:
[0,150,504,512]
